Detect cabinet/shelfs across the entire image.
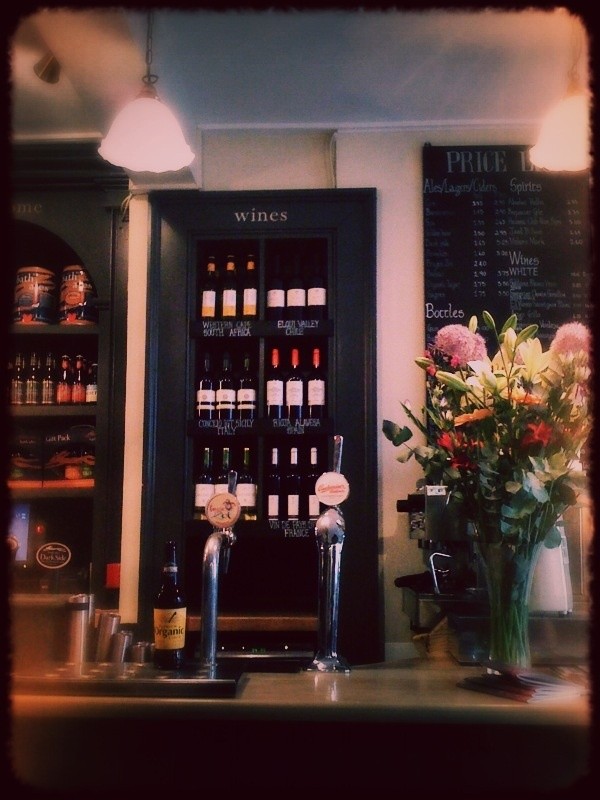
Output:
[8,188,376,662]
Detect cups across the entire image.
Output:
[58,266,99,327]
[12,266,55,325]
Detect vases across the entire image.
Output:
[475,540,541,681]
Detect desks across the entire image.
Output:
[12,668,589,800]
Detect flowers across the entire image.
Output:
[382,311,590,669]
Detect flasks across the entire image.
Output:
[67,594,90,664]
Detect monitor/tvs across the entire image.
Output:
[7,499,38,569]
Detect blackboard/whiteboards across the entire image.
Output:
[422,141,596,447]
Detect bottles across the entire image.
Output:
[264,348,285,428]
[200,256,219,319]
[284,347,306,427]
[196,358,258,427]
[265,254,327,321]
[193,448,259,527]
[306,346,328,426]
[263,446,325,521]
[242,255,260,321]
[0,352,99,404]
[220,255,242,320]
[153,541,187,671]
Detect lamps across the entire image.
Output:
[97,8,195,174]
[529,17,592,171]
[33,51,60,84]
